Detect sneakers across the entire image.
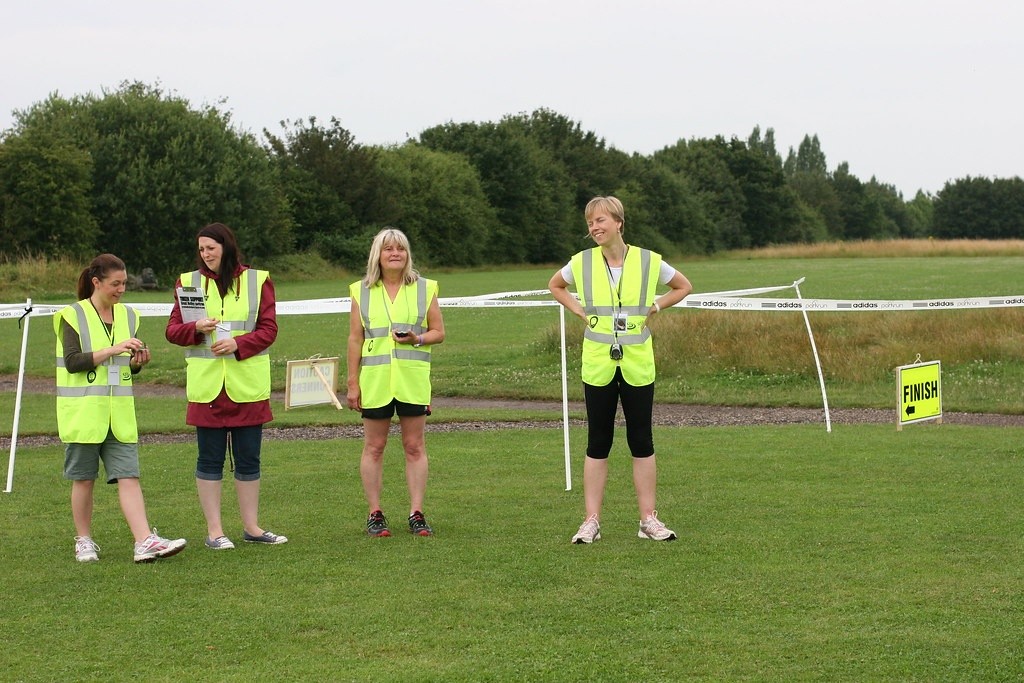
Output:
[409,510,431,535]
[244,530,288,546]
[572,521,601,543]
[364,510,390,537]
[638,518,676,541]
[136,536,186,563]
[204,537,234,549]
[74,537,98,562]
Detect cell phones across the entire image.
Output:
[395,332,408,338]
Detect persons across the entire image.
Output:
[164,222,290,550]
[345,228,447,541]
[50,251,189,568]
[547,196,693,546]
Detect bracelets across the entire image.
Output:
[413,335,424,348]
[652,300,660,313]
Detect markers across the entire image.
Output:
[205,320,230,332]
